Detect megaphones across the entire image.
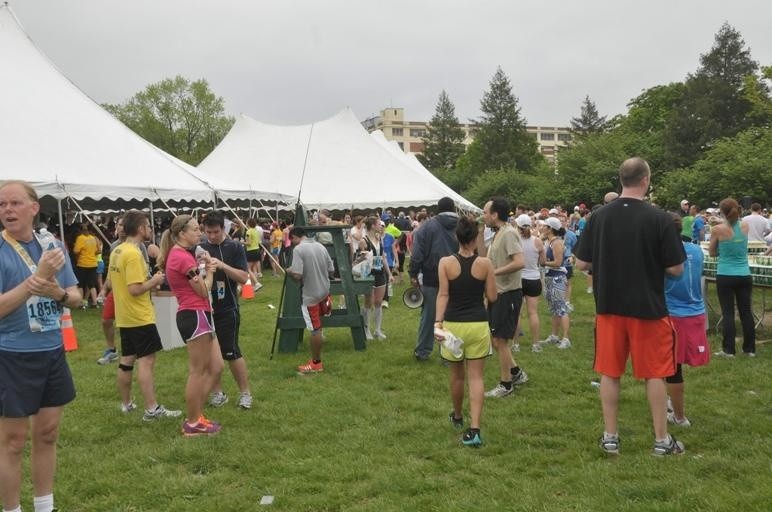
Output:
[403,286,425,309]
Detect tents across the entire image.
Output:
[0,0,483,272]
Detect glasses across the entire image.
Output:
[684,203,688,205]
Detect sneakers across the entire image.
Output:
[716,351,736,357]
[585,285,592,292]
[253,283,265,292]
[97,348,121,367]
[257,273,263,277]
[364,326,372,340]
[323,296,333,317]
[297,359,324,373]
[373,331,388,340]
[122,392,253,436]
[382,300,389,307]
[743,351,756,356]
[449,334,690,457]
[91,304,99,308]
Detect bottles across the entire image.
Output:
[387,280,393,296]
[417,268,423,286]
[196,244,208,277]
[436,324,463,359]
[40,228,57,253]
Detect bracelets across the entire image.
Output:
[58,291,68,305]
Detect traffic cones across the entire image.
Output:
[59,307,80,351]
[240,274,255,299]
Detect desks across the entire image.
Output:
[151,289,185,351]
[700,276,772,335]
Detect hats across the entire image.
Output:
[381,214,390,221]
[705,208,716,215]
[381,221,385,227]
[515,203,586,230]
[681,200,689,208]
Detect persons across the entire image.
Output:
[34,189,767,442]
[0,179,84,512]
[575,156,687,457]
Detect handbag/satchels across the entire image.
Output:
[352,250,373,279]
